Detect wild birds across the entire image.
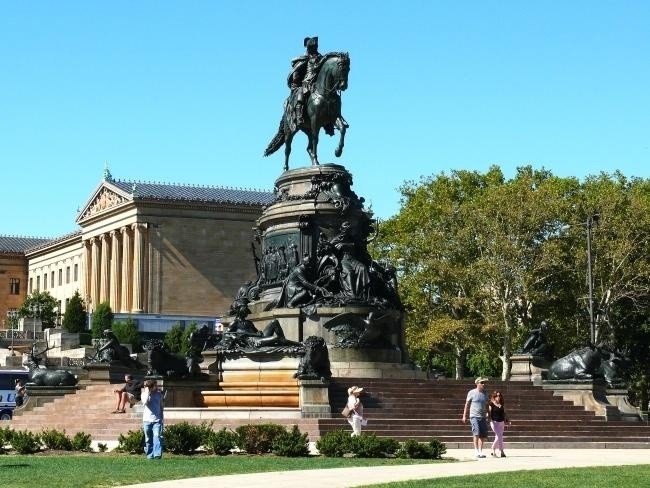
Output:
[321,311,395,346]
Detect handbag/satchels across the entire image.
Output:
[340,405,353,418]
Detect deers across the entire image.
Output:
[17,330,81,394]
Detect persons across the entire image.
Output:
[13,378,26,407]
[521,319,555,359]
[342,385,366,437]
[110,373,141,414]
[98,329,138,370]
[486,390,512,458]
[225,304,307,348]
[140,378,165,461]
[232,220,399,314]
[462,376,491,458]
[292,35,335,137]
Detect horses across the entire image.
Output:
[262,50,351,171]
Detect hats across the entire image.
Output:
[474,376,490,385]
[348,385,363,395]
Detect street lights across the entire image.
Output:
[7,310,21,353]
[28,302,46,349]
[586,214,601,344]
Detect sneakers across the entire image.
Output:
[475,451,506,458]
[111,407,126,414]
